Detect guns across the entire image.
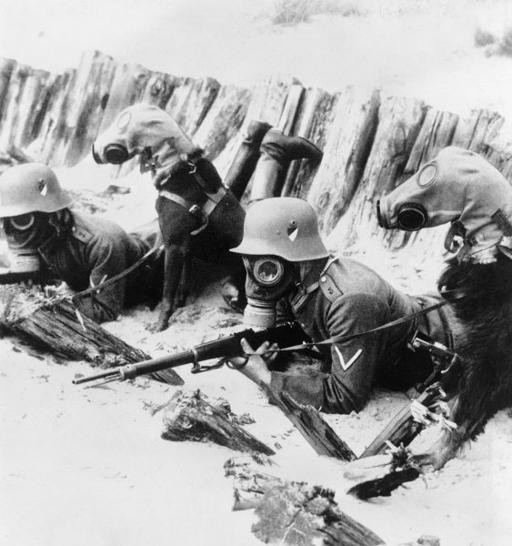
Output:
[0,268,90,291]
[72,320,313,390]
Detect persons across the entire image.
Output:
[226,197,469,414]
[0,120,324,325]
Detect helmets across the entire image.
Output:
[0,163,73,219]
[229,197,329,262]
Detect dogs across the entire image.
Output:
[377,146,511,472]
[88,101,247,334]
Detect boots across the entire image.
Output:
[223,120,323,209]
